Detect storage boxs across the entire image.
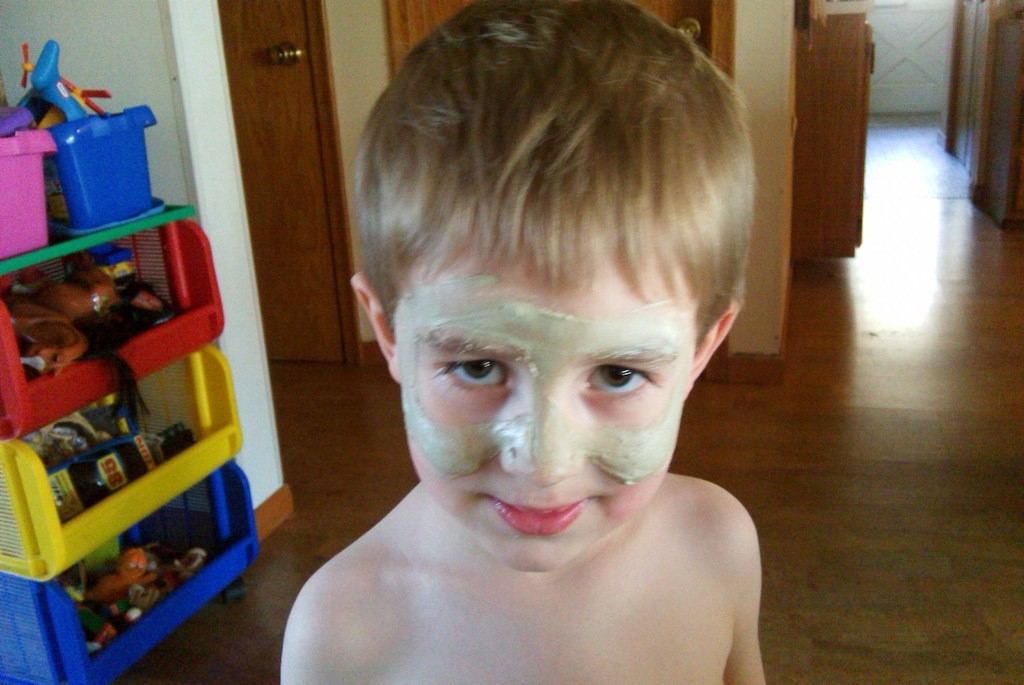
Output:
[43,104,166,238]
[0,128,58,260]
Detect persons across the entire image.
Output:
[279,0,765,685]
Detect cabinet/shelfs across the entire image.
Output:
[792,13,875,258]
[0,205,259,685]
